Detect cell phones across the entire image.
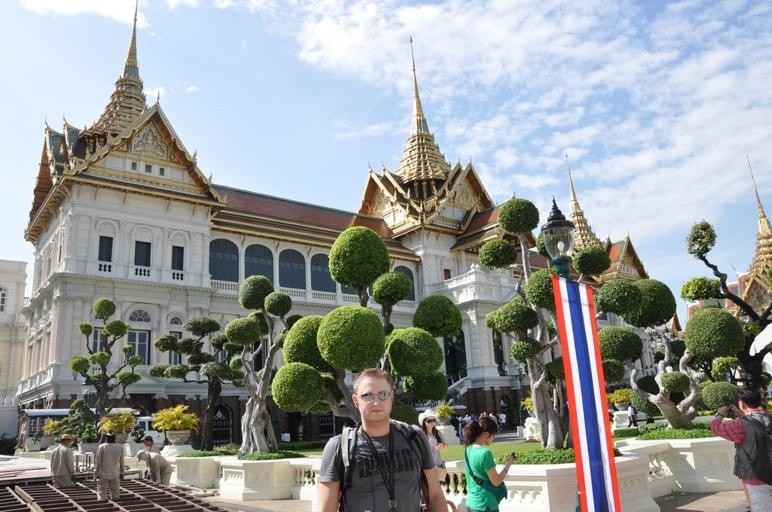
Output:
[508,451,515,458]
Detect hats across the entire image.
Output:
[141,435,152,441]
[418,409,437,426]
[104,431,116,437]
[137,450,145,462]
[61,433,72,440]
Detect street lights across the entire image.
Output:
[539,196,596,512]
[515,366,527,426]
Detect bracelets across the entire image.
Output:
[715,413,724,418]
[504,469,508,474]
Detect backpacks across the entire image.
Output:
[747,416,771,486]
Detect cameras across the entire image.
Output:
[729,407,732,413]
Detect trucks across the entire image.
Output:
[16,405,166,450]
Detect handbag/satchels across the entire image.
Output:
[483,478,508,503]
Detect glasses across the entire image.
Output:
[361,391,390,401]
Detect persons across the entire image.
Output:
[464,417,516,512]
[446,410,507,444]
[627,403,639,427]
[316,367,449,512]
[50,434,76,490]
[136,450,173,487]
[139,435,160,482]
[92,429,125,501]
[710,389,772,512]
[417,409,448,481]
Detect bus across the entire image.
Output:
[414,398,469,420]
